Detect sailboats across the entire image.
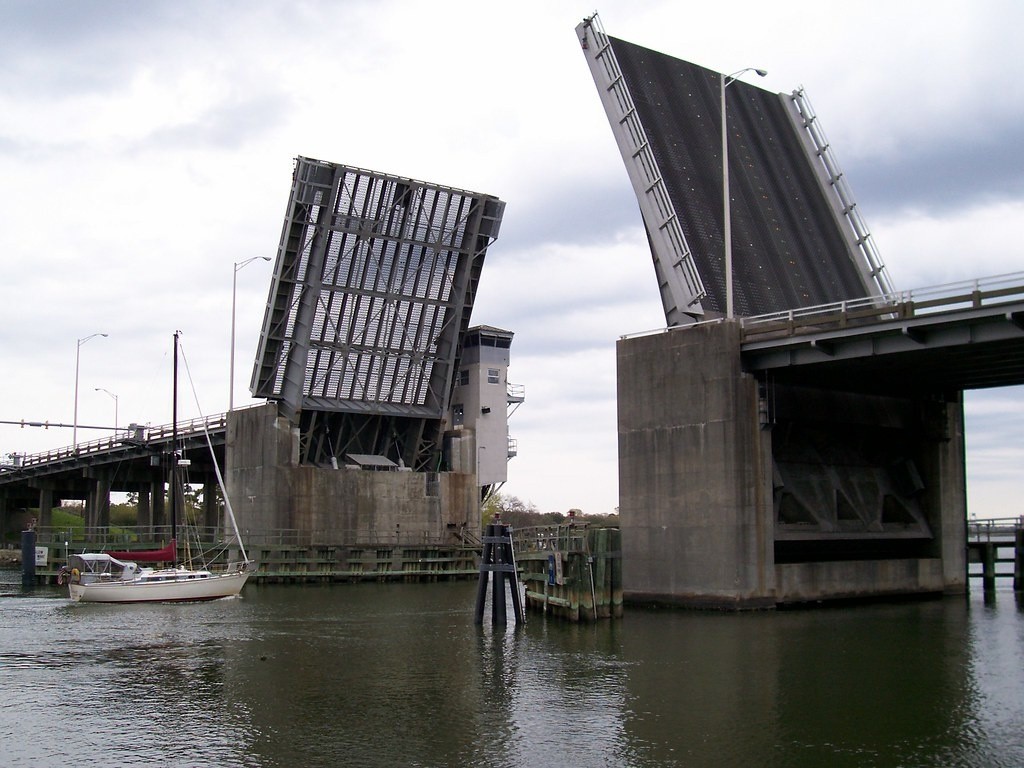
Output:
[65,329,255,602]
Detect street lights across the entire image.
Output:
[72,333,109,454]
[721,68,768,318]
[230,256,272,412]
[94,389,118,447]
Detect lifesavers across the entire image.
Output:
[71,568,81,582]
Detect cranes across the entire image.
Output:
[0,420,164,443]
